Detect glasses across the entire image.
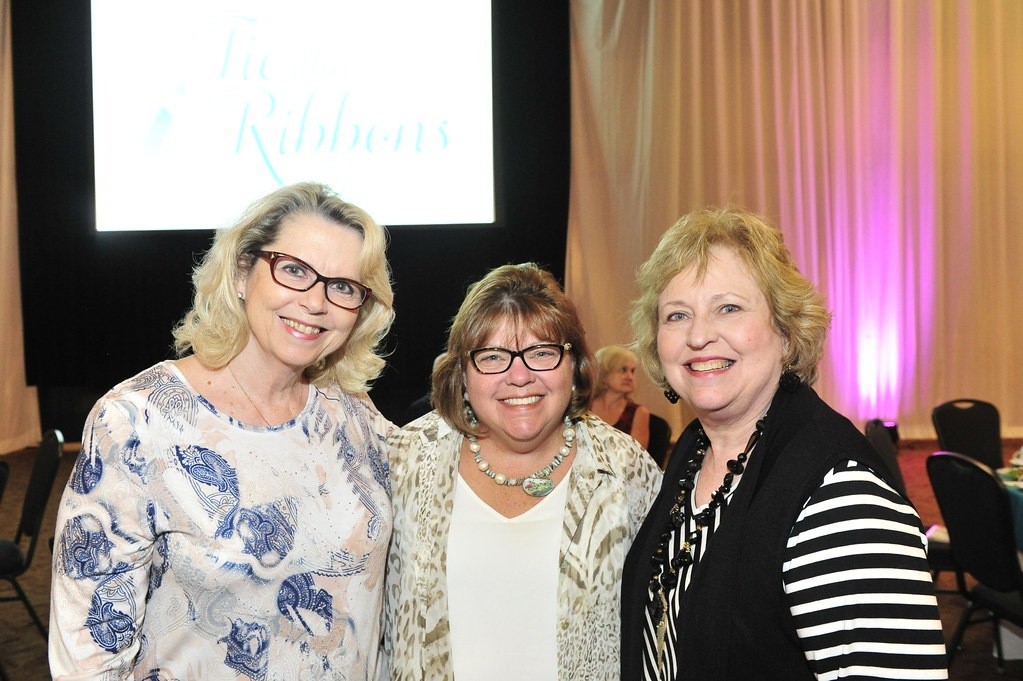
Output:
[247,247,373,312]
[462,340,575,376]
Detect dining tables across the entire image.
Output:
[988,464,1023,557]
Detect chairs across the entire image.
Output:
[930,398,1006,472]
[0,429,66,645]
[865,417,971,600]
[921,451,1023,670]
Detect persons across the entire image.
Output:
[47,181,404,680]
[619,203,949,681]
[587,345,650,450]
[385,261,665,681]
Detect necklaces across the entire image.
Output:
[227,362,306,426]
[466,415,576,497]
[647,414,773,627]
